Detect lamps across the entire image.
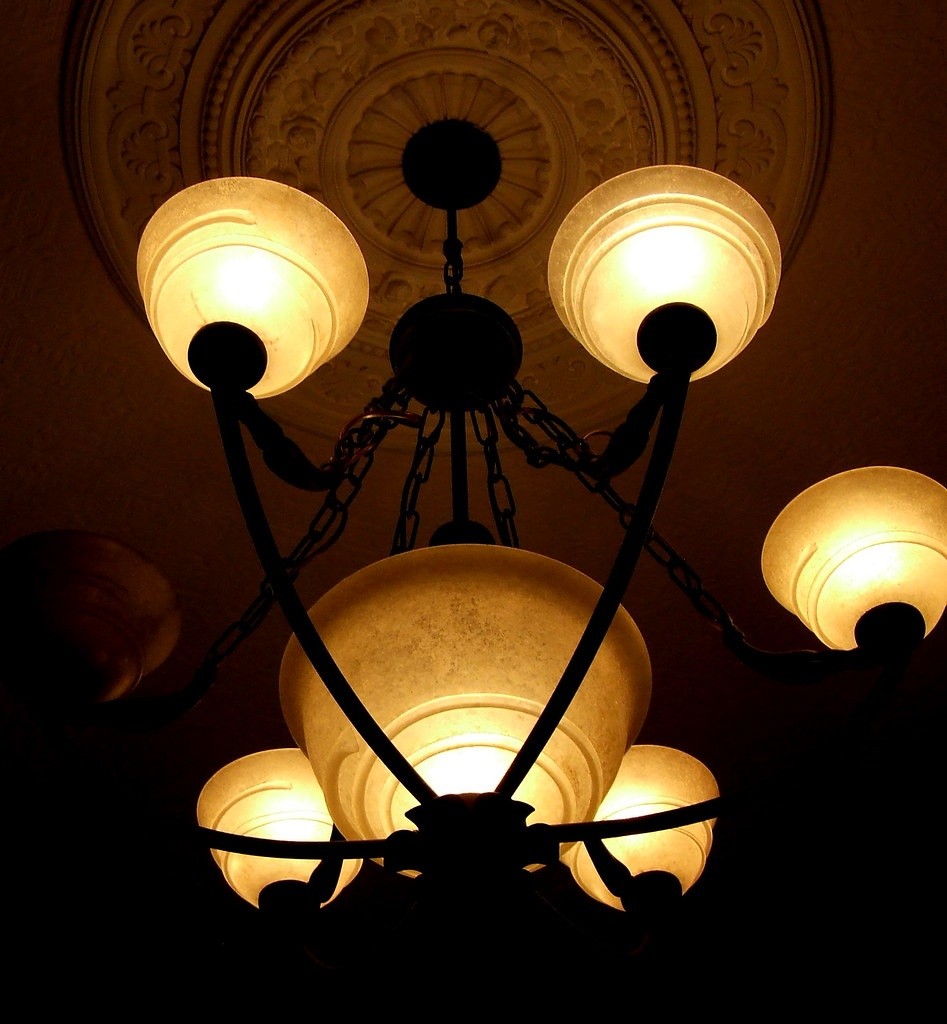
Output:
[1,116,947,981]
